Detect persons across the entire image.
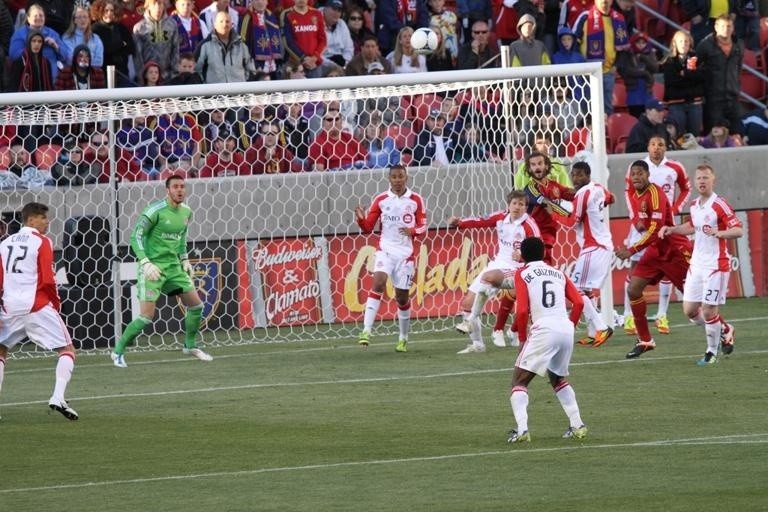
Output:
[613,159,736,358]
[657,165,745,367]
[505,236,590,445]
[0,0,768,188]
[448,189,544,355]
[489,152,577,348]
[354,163,430,354]
[0,201,82,422]
[622,134,693,335]
[543,162,614,349]
[108,173,215,369]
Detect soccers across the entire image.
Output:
[411,27,438,57]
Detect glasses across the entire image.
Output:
[474,30,486,34]
[94,142,109,146]
[552,81,566,87]
[264,130,276,136]
[326,117,339,121]
[351,16,362,21]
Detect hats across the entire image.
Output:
[368,62,384,72]
[645,97,664,112]
[427,110,443,120]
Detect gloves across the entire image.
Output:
[182,259,195,278]
[142,262,162,281]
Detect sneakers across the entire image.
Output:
[563,425,587,440]
[508,431,531,443]
[576,326,613,347]
[357,331,369,345]
[182,346,211,360]
[697,351,719,365]
[48,397,78,420]
[656,317,670,334]
[395,338,407,352]
[721,323,735,354]
[110,350,127,367]
[455,321,520,355]
[626,339,656,358]
[625,315,637,335]
